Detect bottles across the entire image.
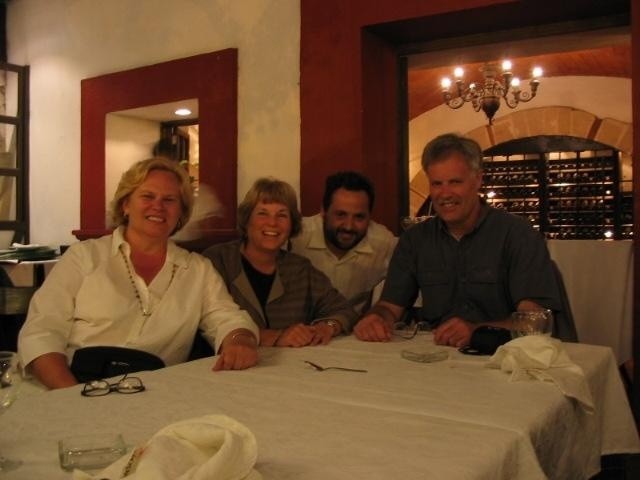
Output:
[482,161,617,239]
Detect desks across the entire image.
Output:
[1,257,64,315]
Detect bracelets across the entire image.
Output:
[229,331,256,342]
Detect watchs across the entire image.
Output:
[323,319,338,337]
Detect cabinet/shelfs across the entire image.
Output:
[473,151,620,240]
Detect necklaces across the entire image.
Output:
[118,246,180,318]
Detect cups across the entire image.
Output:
[401,215,431,232]
[510,309,555,341]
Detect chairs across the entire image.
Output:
[176,237,238,356]
[551,259,575,341]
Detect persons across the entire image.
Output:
[354,135,556,347]
[288,174,400,313]
[200,178,362,346]
[13,155,259,392]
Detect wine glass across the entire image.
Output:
[0,352,27,472]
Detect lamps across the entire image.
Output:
[438,59,544,126]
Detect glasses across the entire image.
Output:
[389,321,434,340]
[80,372,146,398]
